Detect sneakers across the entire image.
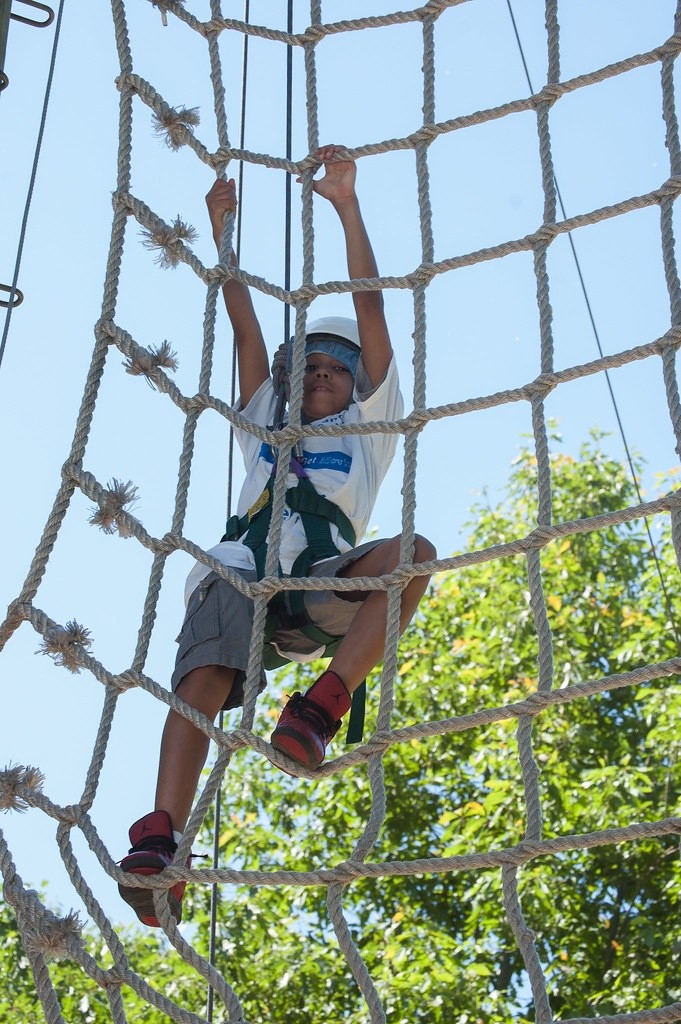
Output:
[112,809,208,927]
[272,670,353,771]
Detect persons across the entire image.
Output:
[119,146,438,926]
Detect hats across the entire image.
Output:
[295,314,369,382]
